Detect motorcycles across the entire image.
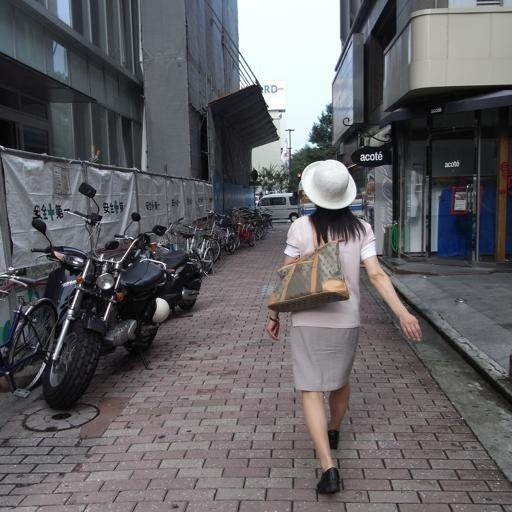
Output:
[29,181,204,414]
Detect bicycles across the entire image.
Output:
[169,204,273,280]
[0,266,60,400]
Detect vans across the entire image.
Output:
[256,192,299,222]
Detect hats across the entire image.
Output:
[301,159,357,211]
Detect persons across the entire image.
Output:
[266,159,422,494]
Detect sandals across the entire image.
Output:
[328,429,340,449]
[318,467,340,493]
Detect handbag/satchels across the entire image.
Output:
[267,240,349,313]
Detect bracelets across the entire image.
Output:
[270,317,279,322]
